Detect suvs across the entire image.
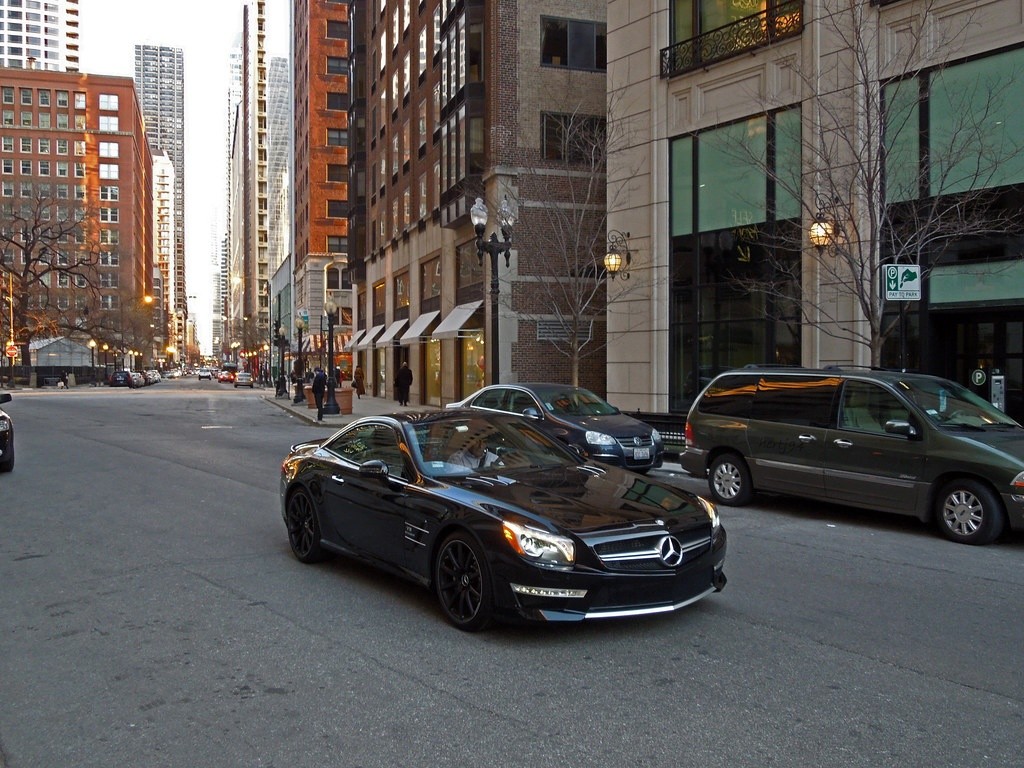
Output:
[234,373,253,388]
[678,363,1024,543]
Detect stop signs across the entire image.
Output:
[6,346,18,357]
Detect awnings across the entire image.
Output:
[432,300,484,339]
[400,310,440,345]
[376,318,409,348]
[344,329,366,348]
[358,324,385,349]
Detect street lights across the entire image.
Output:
[277,322,288,396]
[167,347,176,371]
[240,345,269,387]
[90,339,143,381]
[320,300,341,414]
[471,195,516,385]
[291,317,307,406]
[223,316,250,373]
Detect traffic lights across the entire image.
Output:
[273,321,281,337]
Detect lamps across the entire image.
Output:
[808,193,842,257]
[604,230,632,281]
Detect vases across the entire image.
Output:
[304,386,317,409]
[334,388,353,413]
[323,390,327,402]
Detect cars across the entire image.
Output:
[218,371,234,383]
[161,367,221,380]
[444,383,665,475]
[280,408,728,634]
[109,370,161,388]
[0,393,14,473]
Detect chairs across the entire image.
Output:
[843,392,881,430]
[882,392,909,421]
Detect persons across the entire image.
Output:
[354,366,365,399]
[395,362,413,406]
[59,370,69,389]
[312,367,327,422]
[334,365,343,388]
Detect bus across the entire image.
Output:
[221,363,238,373]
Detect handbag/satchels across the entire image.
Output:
[351,379,359,388]
[58,379,65,386]
